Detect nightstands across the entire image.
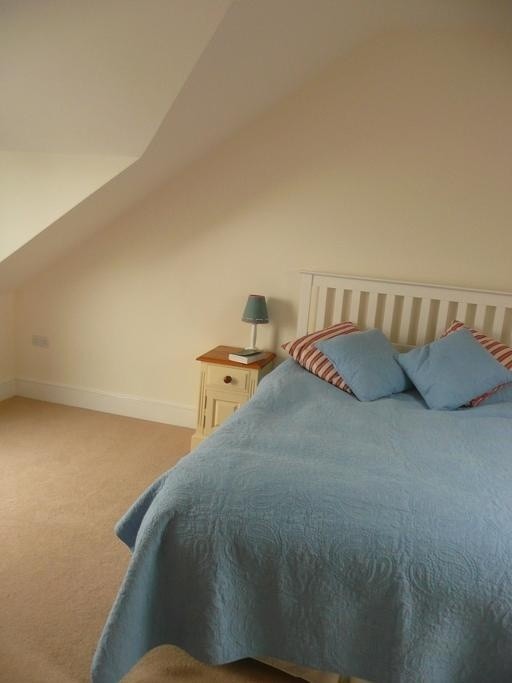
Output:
[190,344,276,454]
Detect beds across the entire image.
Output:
[139,271,512,683]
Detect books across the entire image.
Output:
[227,348,269,365]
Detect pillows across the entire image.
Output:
[276,320,512,411]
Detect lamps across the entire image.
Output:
[242,294,269,352]
